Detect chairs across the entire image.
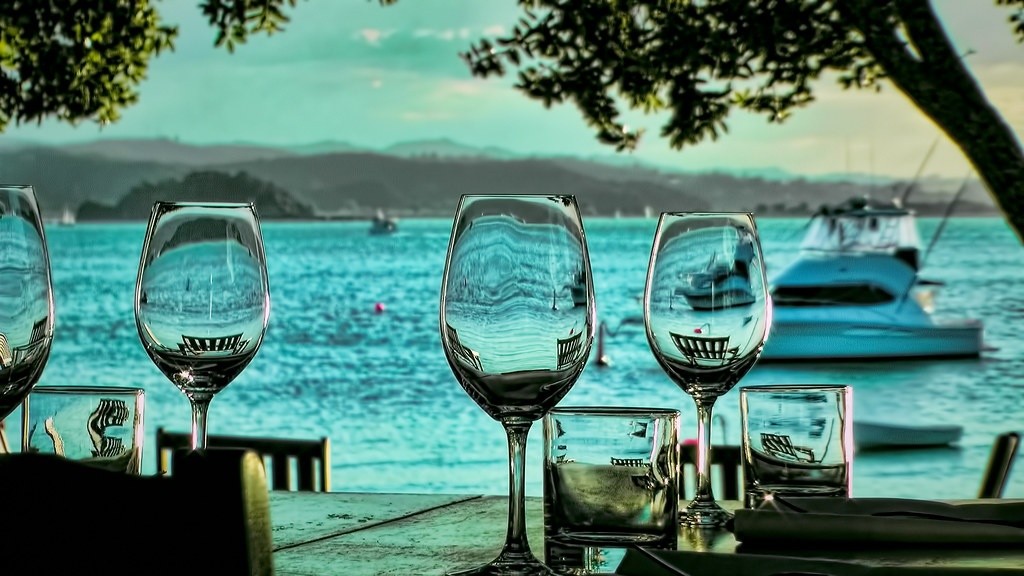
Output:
[154,425,332,495]
[659,442,744,503]
[975,431,1021,499]
[0,447,276,576]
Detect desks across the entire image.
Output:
[268,489,1023,576]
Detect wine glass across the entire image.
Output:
[643,212,770,533]
[133,201,270,452]
[440,191,595,576]
[0,183,56,424]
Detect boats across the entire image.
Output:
[561,257,589,307]
[60,208,76,227]
[368,207,401,237]
[756,133,1002,365]
[674,262,756,312]
[852,414,966,450]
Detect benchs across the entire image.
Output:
[609,456,644,468]
[10,315,49,378]
[760,432,817,463]
[445,322,484,372]
[176,332,252,356]
[557,329,584,370]
[668,331,742,367]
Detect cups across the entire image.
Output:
[22,383,144,479]
[543,408,680,576]
[739,385,853,510]
